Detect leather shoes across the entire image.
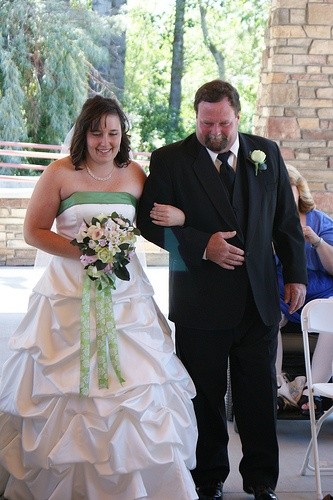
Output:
[199,481,222,500]
[254,487,278,500]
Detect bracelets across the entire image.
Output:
[310,237,327,249]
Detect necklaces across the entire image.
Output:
[82,159,118,180]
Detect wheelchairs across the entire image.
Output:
[225,339,333,433]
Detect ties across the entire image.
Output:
[217,150,236,207]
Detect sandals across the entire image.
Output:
[297,395,326,417]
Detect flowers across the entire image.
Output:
[247,149,268,176]
[70,211,142,293]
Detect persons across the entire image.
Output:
[136,80,307,500]
[272,162,332,417]
[1,96,198,500]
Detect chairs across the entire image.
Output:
[300,298,333,500]
